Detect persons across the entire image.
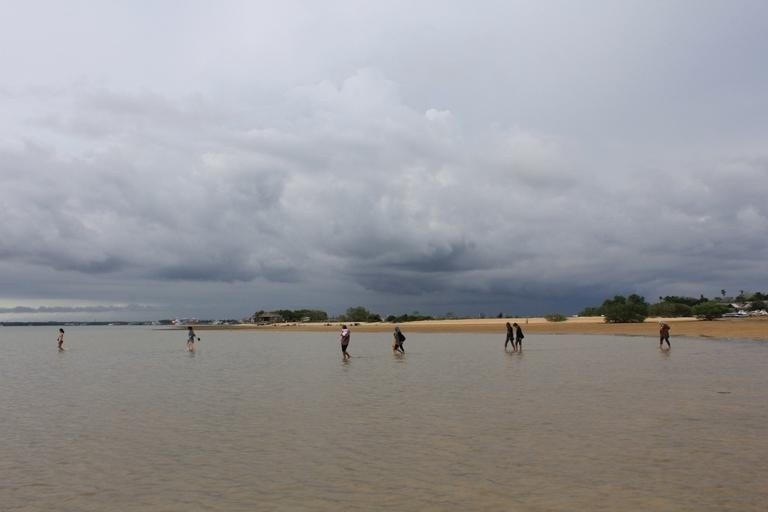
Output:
[339,334,351,361]
[658,320,671,349]
[185,327,200,353]
[392,333,402,355]
[512,322,524,354]
[341,326,350,338]
[56,328,64,351]
[504,322,515,352]
[395,326,406,354]
